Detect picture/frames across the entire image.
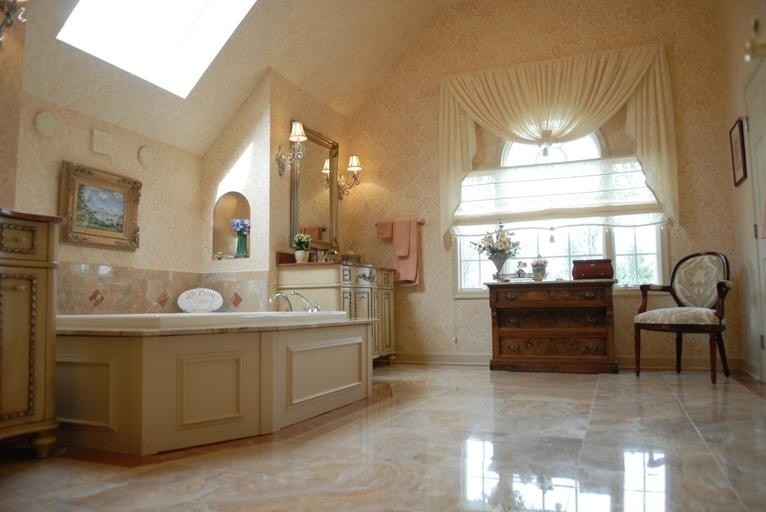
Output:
[55,157,143,255]
[727,116,748,188]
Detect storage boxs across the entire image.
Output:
[568,256,616,283]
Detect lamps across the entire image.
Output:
[320,156,332,189]
[336,153,363,201]
[273,119,309,179]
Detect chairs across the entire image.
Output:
[629,247,734,386]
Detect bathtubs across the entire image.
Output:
[52,309,353,329]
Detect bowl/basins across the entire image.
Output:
[308,240,332,250]
[342,254,361,264]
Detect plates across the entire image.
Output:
[177,288,223,312]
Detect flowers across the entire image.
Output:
[464,217,522,258]
[289,233,313,252]
[230,216,253,238]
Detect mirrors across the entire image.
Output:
[286,117,340,255]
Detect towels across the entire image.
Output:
[375,217,423,291]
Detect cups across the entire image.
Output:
[310,250,324,262]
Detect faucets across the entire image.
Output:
[290,290,319,312]
[268,293,293,312]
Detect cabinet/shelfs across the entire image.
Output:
[0,205,68,456]
[480,276,623,376]
[275,259,372,364]
[367,264,399,368]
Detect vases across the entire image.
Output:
[486,252,508,281]
[293,247,309,265]
[232,230,249,259]
